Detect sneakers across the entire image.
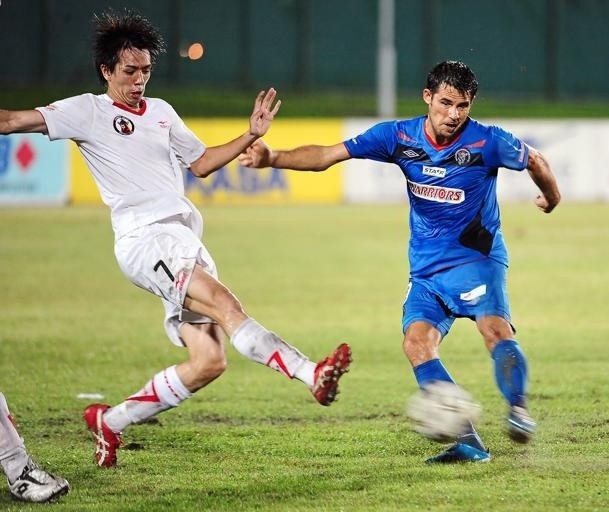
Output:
[307,343,353,406]
[425,443,490,464]
[509,414,533,443]
[7,463,70,503]
[83,403,120,468]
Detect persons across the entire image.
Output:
[0,392,70,502]
[238,60,560,465]
[0,15,351,467]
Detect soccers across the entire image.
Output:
[407,379,482,443]
